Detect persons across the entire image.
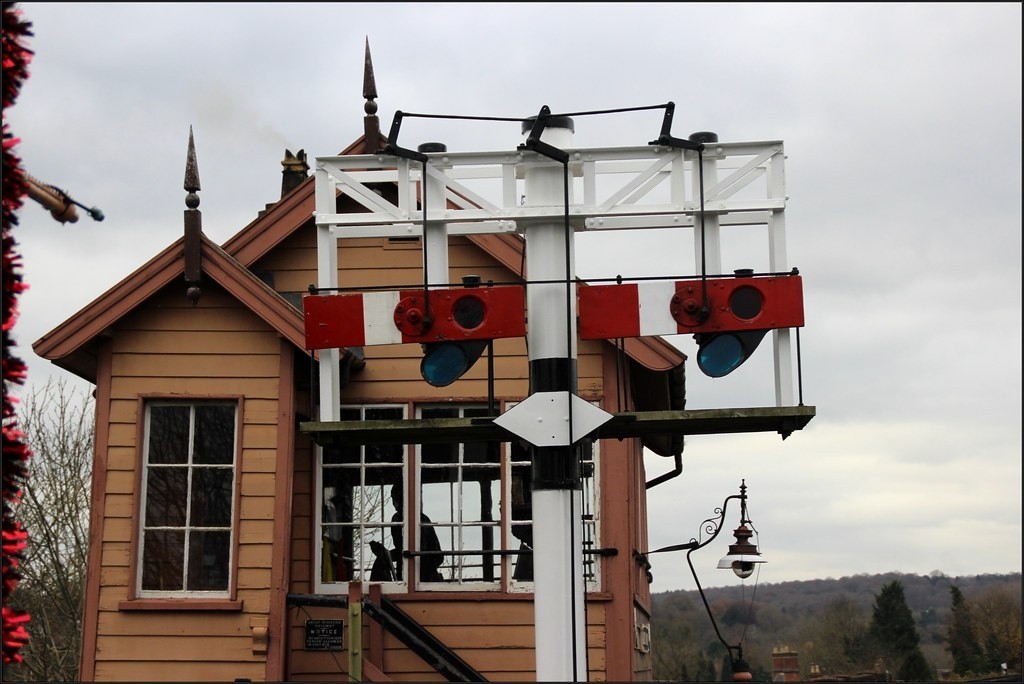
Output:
[386,483,444,581]
[509,479,534,582]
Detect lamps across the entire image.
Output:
[643,480,768,684]
